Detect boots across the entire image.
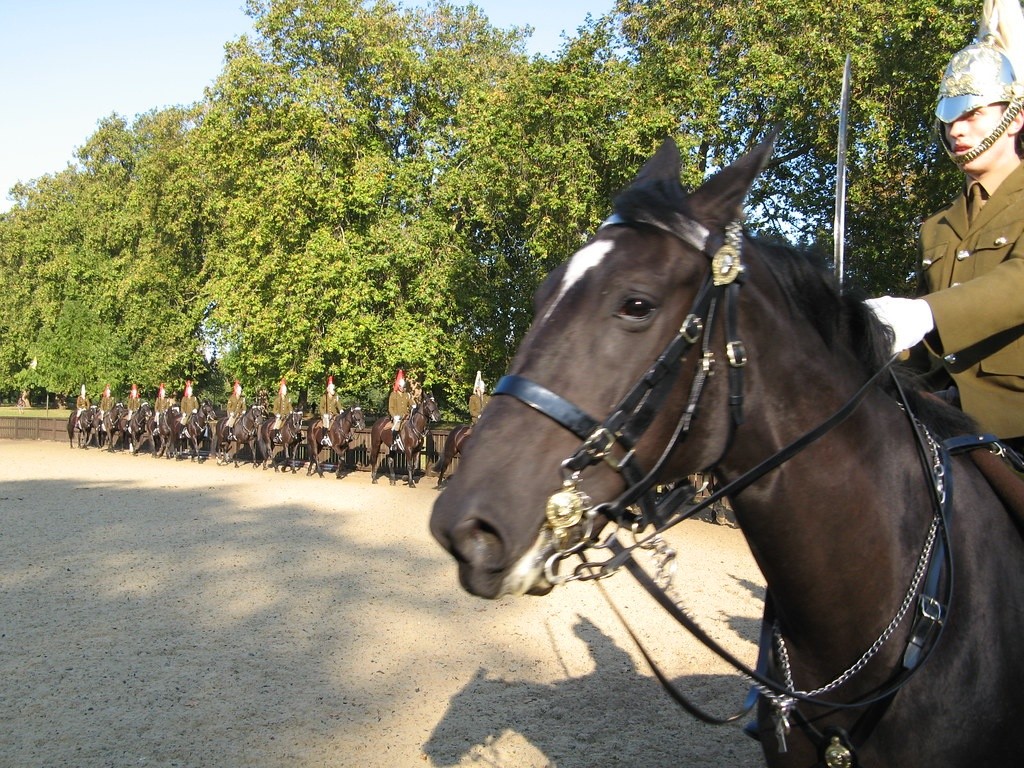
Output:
[321,426,329,445]
[390,430,399,451]
[178,423,185,439]
[126,419,130,427]
[227,427,233,440]
[274,429,279,443]
[154,422,158,435]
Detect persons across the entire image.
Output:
[18,397,26,415]
[742,0,1024,743]
[74,371,494,452]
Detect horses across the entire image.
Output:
[66,390,741,528]
[429,118,1024,768]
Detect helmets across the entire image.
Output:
[474,371,485,394]
[931,0,1024,125]
[327,375,336,393]
[185,381,193,395]
[279,379,288,393]
[233,380,242,394]
[81,384,86,396]
[392,370,407,390]
[159,383,166,397]
[130,383,138,397]
[104,385,111,395]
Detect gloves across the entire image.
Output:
[242,411,246,414]
[101,410,105,414]
[156,412,160,416]
[231,412,235,417]
[193,408,198,413]
[324,414,329,418]
[277,414,281,419]
[863,295,935,356]
[339,409,344,414]
[395,415,400,422]
[182,412,187,416]
[412,405,416,410]
